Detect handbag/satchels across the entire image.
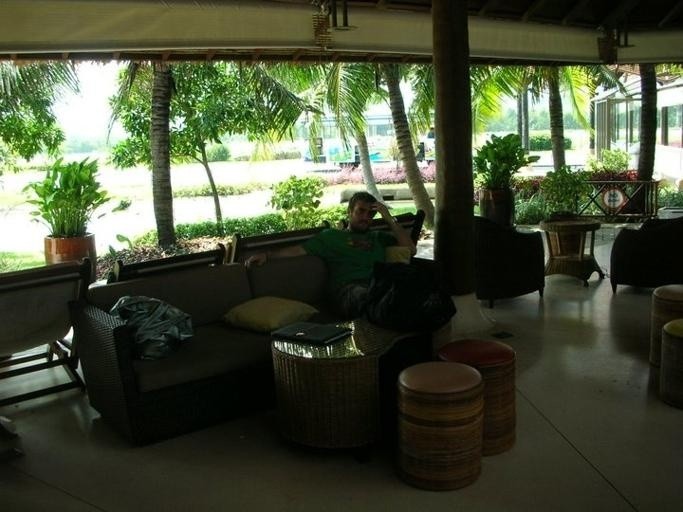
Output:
[109,294,194,362]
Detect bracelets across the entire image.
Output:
[389,217,397,225]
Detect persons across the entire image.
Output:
[244,194,417,320]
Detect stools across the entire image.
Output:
[649,284,683,368]
[659,319,683,409]
[397,361,486,492]
[436,337,516,456]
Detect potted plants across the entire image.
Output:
[472,133,541,227]
[22,155,111,285]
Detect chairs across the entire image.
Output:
[470,215,544,309]
[339,209,426,245]
[610,215,683,294]
[223,220,330,265]
[0,259,90,409]
[106,242,226,284]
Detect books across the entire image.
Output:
[270,321,354,345]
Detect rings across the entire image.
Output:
[381,208,383,210]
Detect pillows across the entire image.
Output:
[244,251,335,325]
[85,263,252,328]
[385,245,411,265]
[222,297,321,334]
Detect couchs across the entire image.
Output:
[67,242,433,447]
